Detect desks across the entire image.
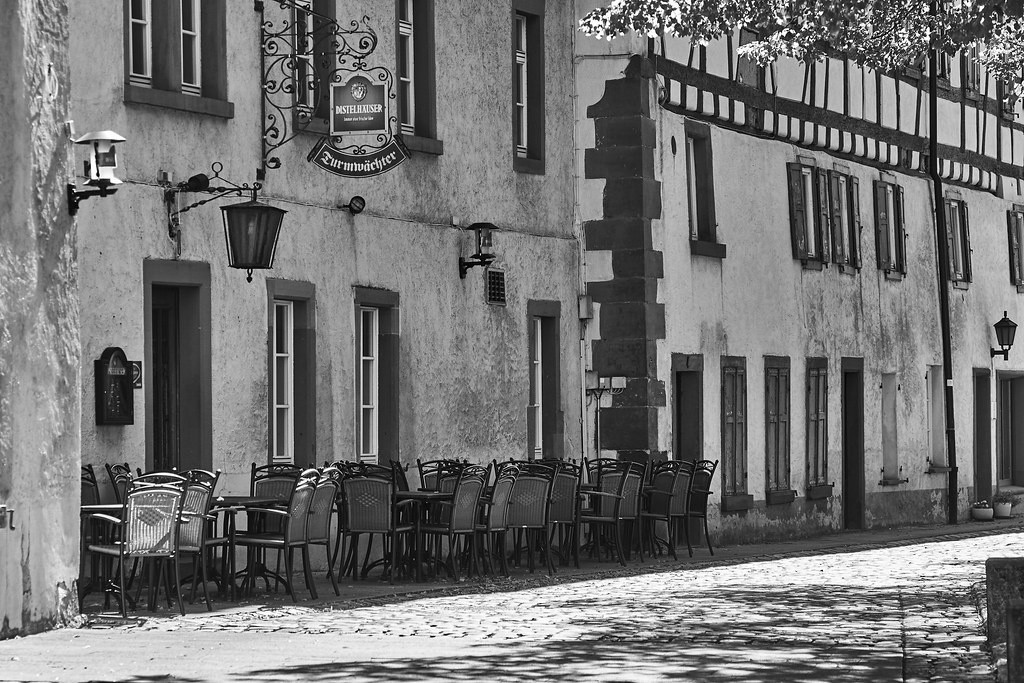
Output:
[81,502,173,612]
[209,496,276,609]
[336,490,454,585]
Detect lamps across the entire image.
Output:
[66,129,125,217]
[991,311,1018,360]
[458,222,499,280]
[166,162,286,283]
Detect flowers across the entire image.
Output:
[971,497,992,509]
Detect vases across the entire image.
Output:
[972,508,993,520]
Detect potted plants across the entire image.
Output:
[992,489,1022,517]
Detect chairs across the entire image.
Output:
[82,447,720,624]
